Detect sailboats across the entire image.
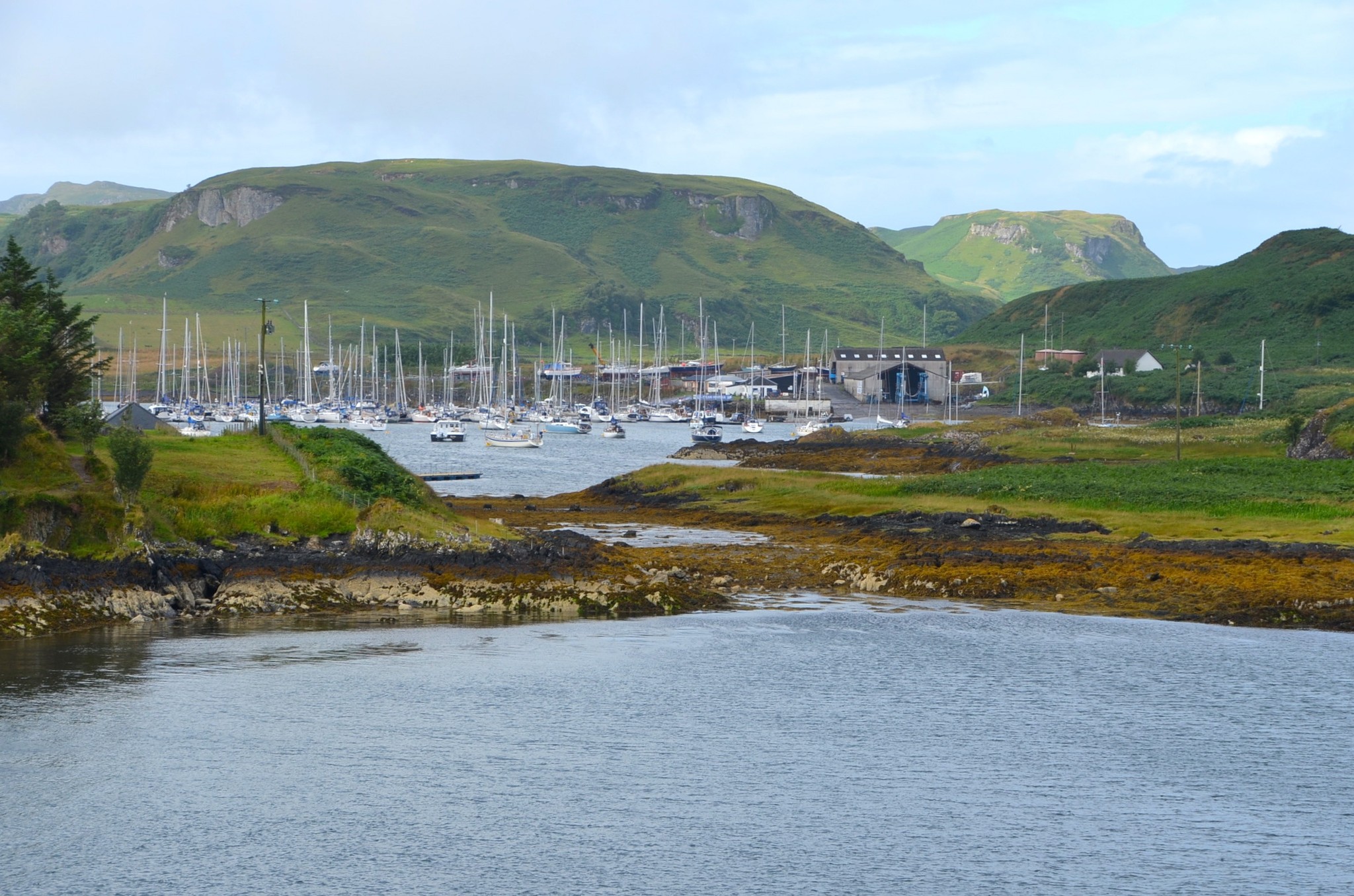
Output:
[690,314,725,444]
[1086,353,1115,428]
[870,315,917,430]
[484,313,543,449]
[789,327,836,437]
[51,289,764,438]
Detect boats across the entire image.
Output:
[429,419,468,442]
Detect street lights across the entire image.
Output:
[252,297,280,435]
